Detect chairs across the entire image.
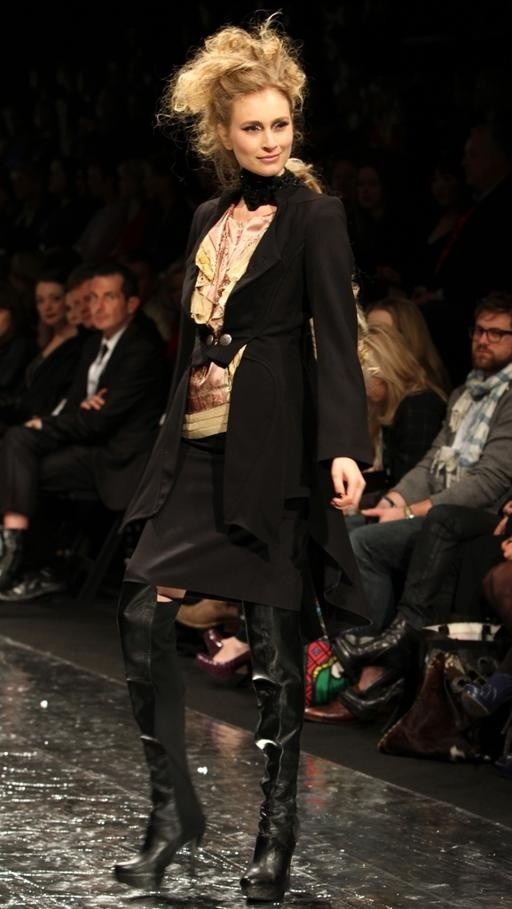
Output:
[66,490,144,600]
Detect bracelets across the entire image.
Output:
[383,495,396,508]
[402,505,415,519]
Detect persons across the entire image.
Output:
[336,500,511,731]
[2,261,186,600]
[113,10,375,904]
[365,297,453,397]
[351,322,446,504]
[176,598,238,628]
[195,612,251,684]
[304,293,510,724]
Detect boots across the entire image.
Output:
[240,603,304,901]
[332,614,418,665]
[342,672,415,720]
[109,580,207,890]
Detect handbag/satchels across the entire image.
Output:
[379,648,511,762]
[305,633,350,703]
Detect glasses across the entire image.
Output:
[470,324,511,343]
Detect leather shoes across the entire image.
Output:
[196,638,252,677]
[1,566,70,603]
[0,531,29,576]
[305,683,364,721]
